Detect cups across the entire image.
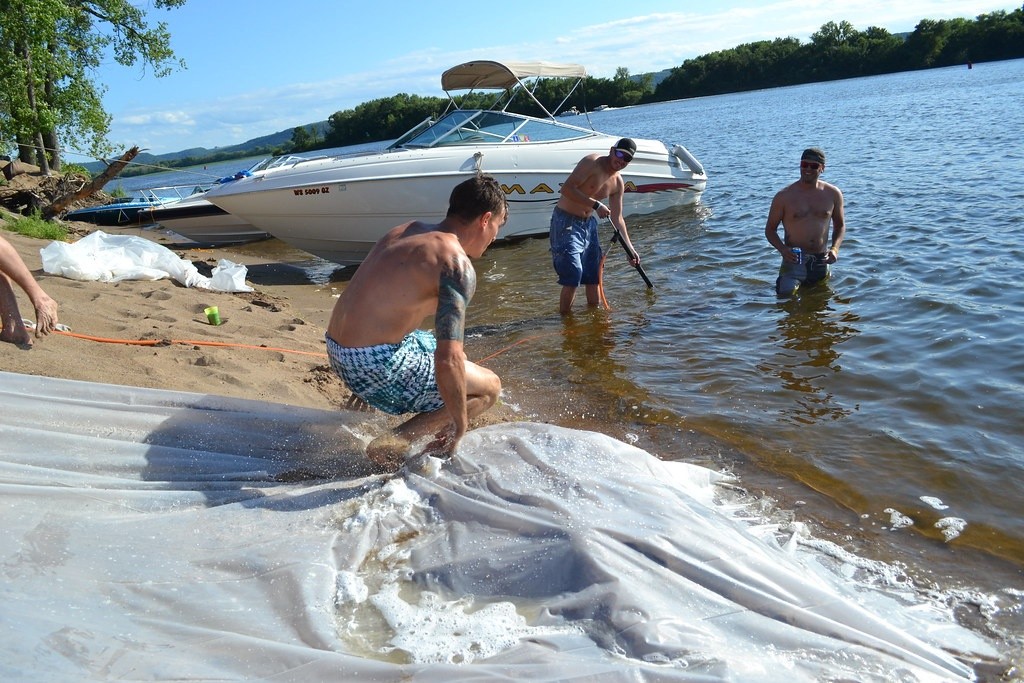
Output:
[204,306,221,325]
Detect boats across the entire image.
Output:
[203,58,707,266]
[134,152,327,247]
[593,104,608,110]
[560,106,580,117]
[62,196,178,224]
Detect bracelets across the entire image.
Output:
[593,202,602,211]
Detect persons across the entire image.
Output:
[1,234,59,350]
[766,150,846,295]
[327,173,509,473]
[550,139,641,314]
